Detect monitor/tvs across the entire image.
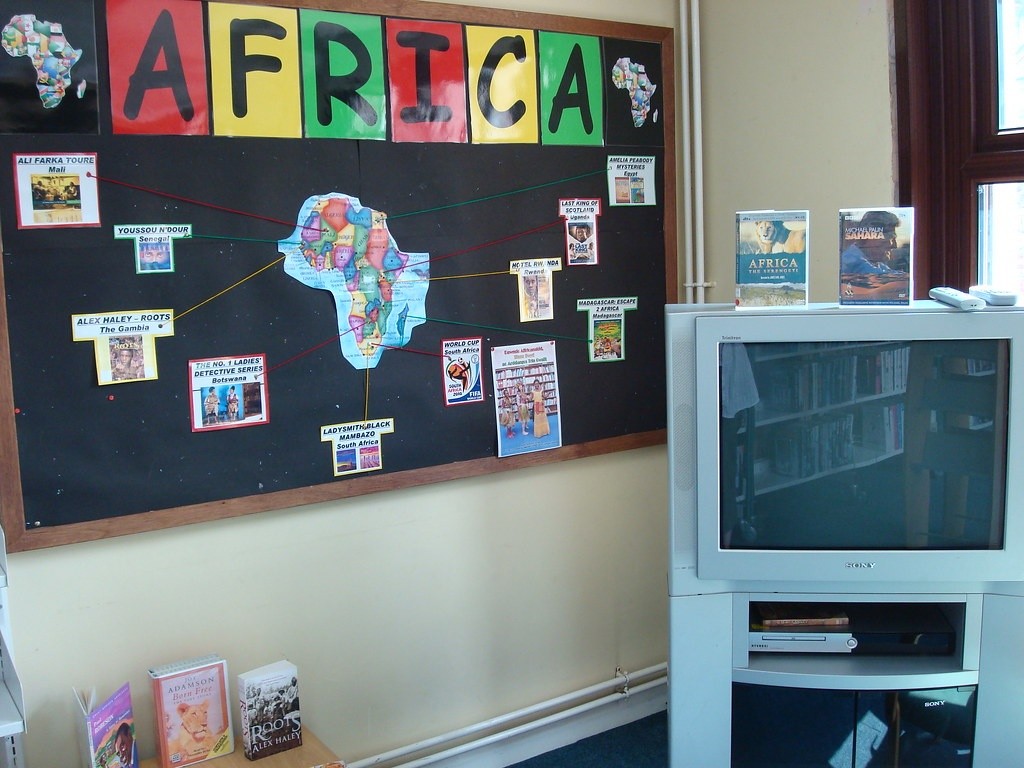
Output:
[663,302,1024,596]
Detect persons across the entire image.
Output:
[98,753,109,768]
[569,242,594,259]
[116,722,139,768]
[841,211,904,274]
[112,349,137,380]
[500,379,553,439]
[524,275,537,297]
[576,227,587,241]
[252,677,298,722]
[226,385,239,420]
[204,387,221,425]
[34,181,78,200]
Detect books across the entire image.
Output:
[762,609,850,627]
[737,345,998,495]
[71,680,140,768]
[735,209,810,312]
[146,654,235,768]
[839,207,914,309]
[237,660,303,761]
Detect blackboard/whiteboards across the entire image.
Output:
[0,0,685,555]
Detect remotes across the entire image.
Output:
[929,287,986,311]
[969,285,1018,306]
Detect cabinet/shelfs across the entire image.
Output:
[717,342,1013,519]
